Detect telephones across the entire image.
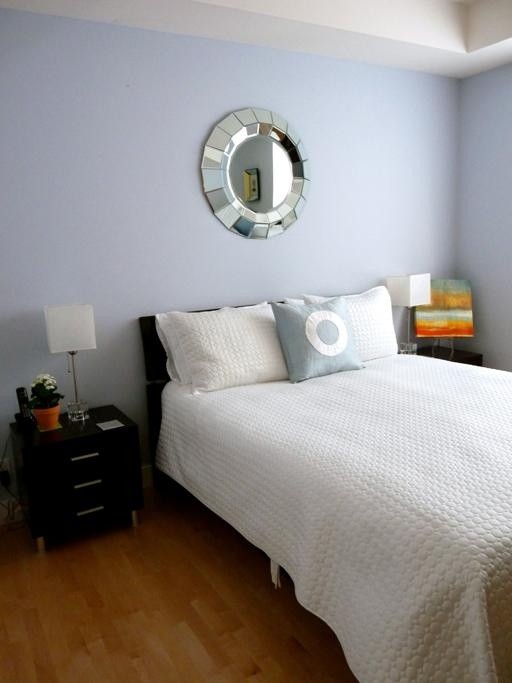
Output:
[14,387,36,427]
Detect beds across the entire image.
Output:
[139,293,510,683]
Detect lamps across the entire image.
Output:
[44,303,97,420]
[386,272,433,354]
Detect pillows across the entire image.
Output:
[154,285,398,396]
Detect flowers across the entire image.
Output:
[30,371,65,405]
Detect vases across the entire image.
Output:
[30,405,61,431]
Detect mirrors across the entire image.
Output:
[199,107,312,240]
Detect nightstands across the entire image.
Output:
[413,343,485,367]
[10,403,144,552]
